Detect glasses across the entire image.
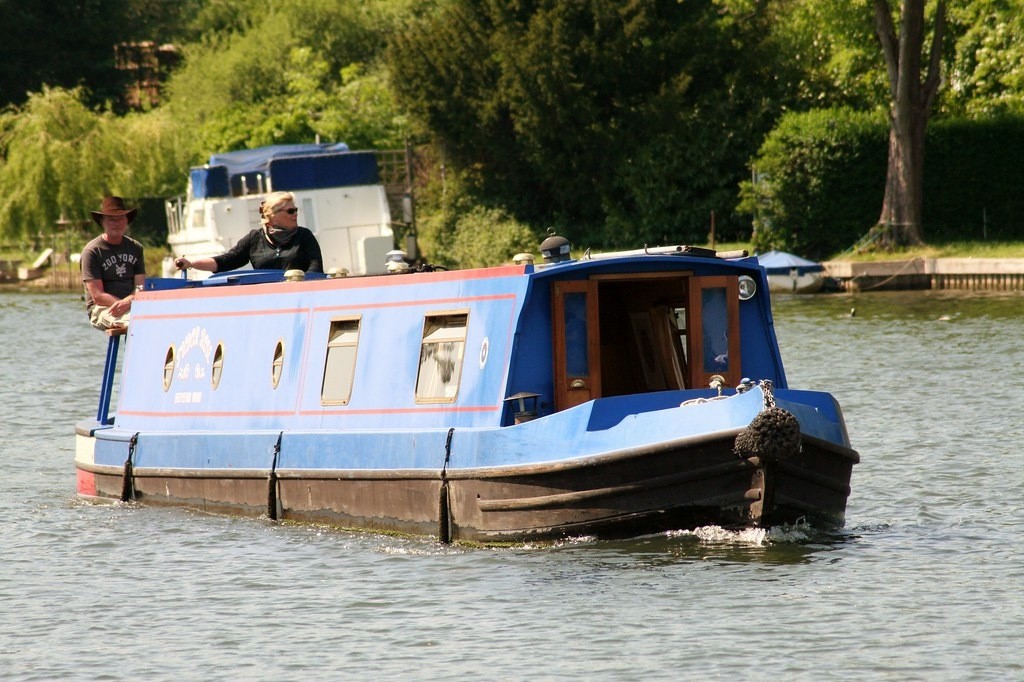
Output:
[279,208,298,215]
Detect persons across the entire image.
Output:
[175,192,324,274]
[80,197,145,331]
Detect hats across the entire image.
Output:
[91,197,138,228]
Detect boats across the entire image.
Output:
[162,126,419,277]
[71,211,861,549]
[753,249,825,293]
